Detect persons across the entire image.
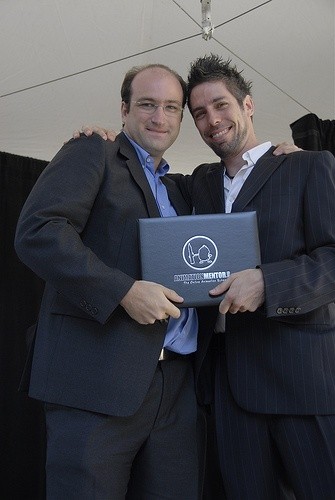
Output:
[63,54,335,500]
[14,65,311,500]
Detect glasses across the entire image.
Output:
[128,101,184,116]
